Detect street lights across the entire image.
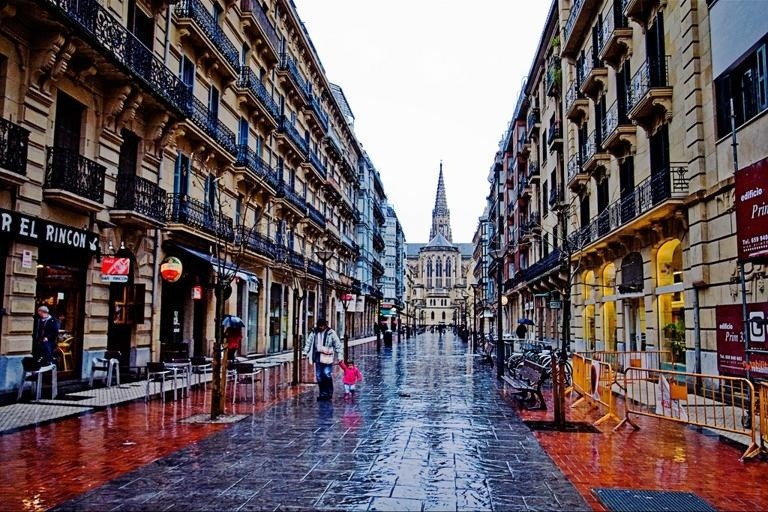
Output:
[449,280,484,359]
[310,247,337,378]
[487,248,508,385]
[373,282,424,359]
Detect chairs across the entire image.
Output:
[88,349,124,390]
[16,352,59,402]
[143,350,292,407]
[57,332,72,376]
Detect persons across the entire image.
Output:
[224,327,242,369]
[340,359,362,400]
[516,322,528,350]
[303,319,342,400]
[37,305,59,366]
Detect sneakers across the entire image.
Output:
[343,392,358,401]
[316,394,333,402]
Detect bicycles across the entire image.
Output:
[507,338,572,387]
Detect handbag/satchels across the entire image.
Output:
[319,352,335,365]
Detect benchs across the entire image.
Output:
[475,341,496,370]
[499,360,551,412]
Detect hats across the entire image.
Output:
[316,318,328,326]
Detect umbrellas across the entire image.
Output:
[517,318,534,328]
[221,316,246,328]
